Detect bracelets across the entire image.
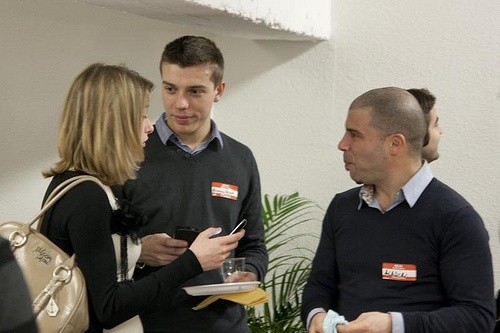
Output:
[136,262,146,270]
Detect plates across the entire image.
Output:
[182,282,261,296]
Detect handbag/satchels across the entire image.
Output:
[1,175,116,333]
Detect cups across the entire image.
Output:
[220,258,245,282]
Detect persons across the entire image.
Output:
[40,61,245,333]
[113,35,268,333]
[405,88,443,164]
[300,87,496,333]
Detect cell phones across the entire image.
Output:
[173,226,201,249]
[229,219,248,236]
